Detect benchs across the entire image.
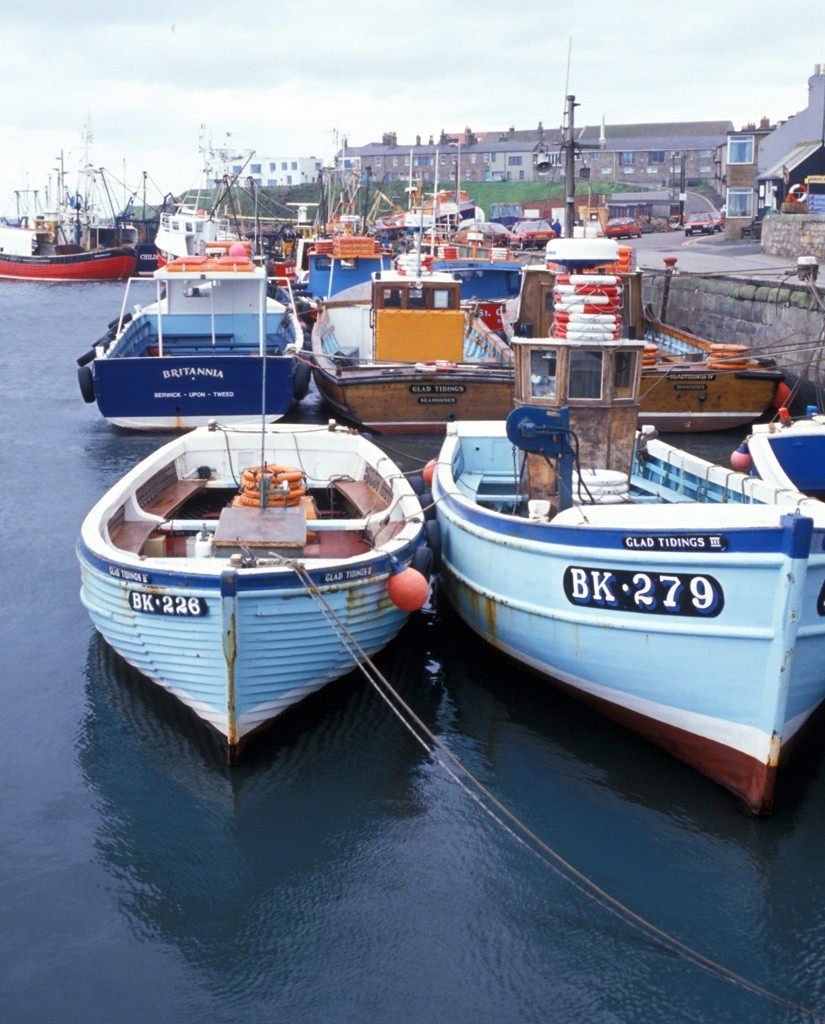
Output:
[125,332,234,358]
[660,349,684,361]
[330,477,405,544]
[629,474,697,505]
[454,472,484,502]
[113,479,206,554]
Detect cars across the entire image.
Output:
[683,213,714,236]
[710,214,724,233]
[605,217,642,239]
[505,220,555,250]
[468,220,511,248]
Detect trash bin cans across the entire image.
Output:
[752,220,761,239]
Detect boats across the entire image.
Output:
[507,318,786,433]
[119,117,522,343]
[309,195,517,435]
[0,113,139,284]
[74,334,427,767]
[92,259,303,434]
[427,94,825,817]
